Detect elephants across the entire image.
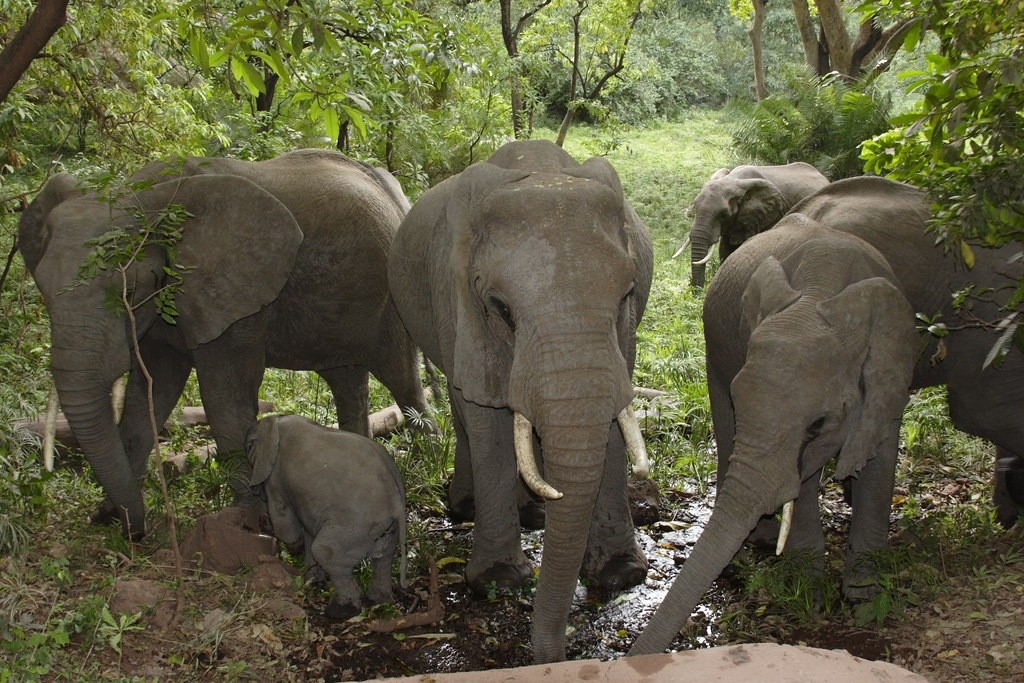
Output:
[20,149,438,622]
[623,160,1024,654]
[388,141,653,665]
